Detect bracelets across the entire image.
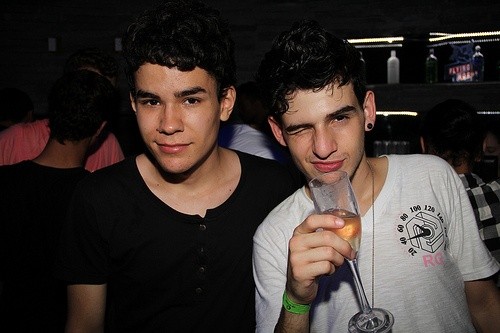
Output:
[282,291,313,315]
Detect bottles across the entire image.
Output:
[386,50,401,85]
[472,45,485,81]
[426,48,439,83]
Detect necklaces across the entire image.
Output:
[370,163,376,308]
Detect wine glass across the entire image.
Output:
[308,172,395,333]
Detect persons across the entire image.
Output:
[0,0,500,333]
